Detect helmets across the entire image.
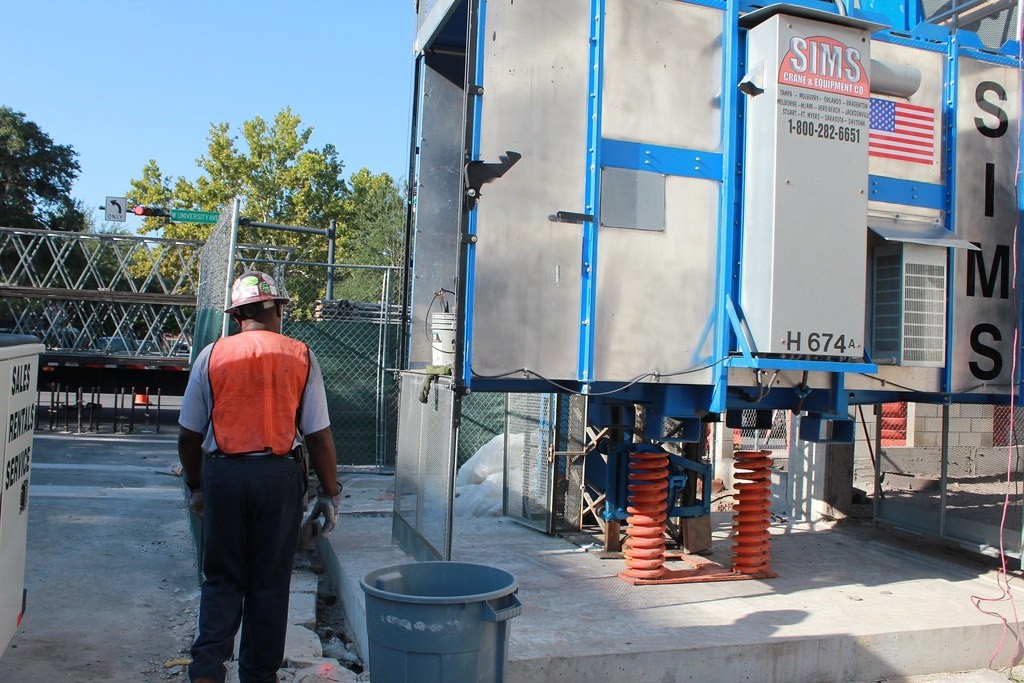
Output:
[224,272,290,315]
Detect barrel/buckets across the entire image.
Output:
[359,560,522,683]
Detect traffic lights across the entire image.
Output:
[133,206,166,217]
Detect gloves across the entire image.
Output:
[309,483,343,538]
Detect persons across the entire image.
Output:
[179,271,344,683]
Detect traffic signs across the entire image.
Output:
[105,195,127,223]
